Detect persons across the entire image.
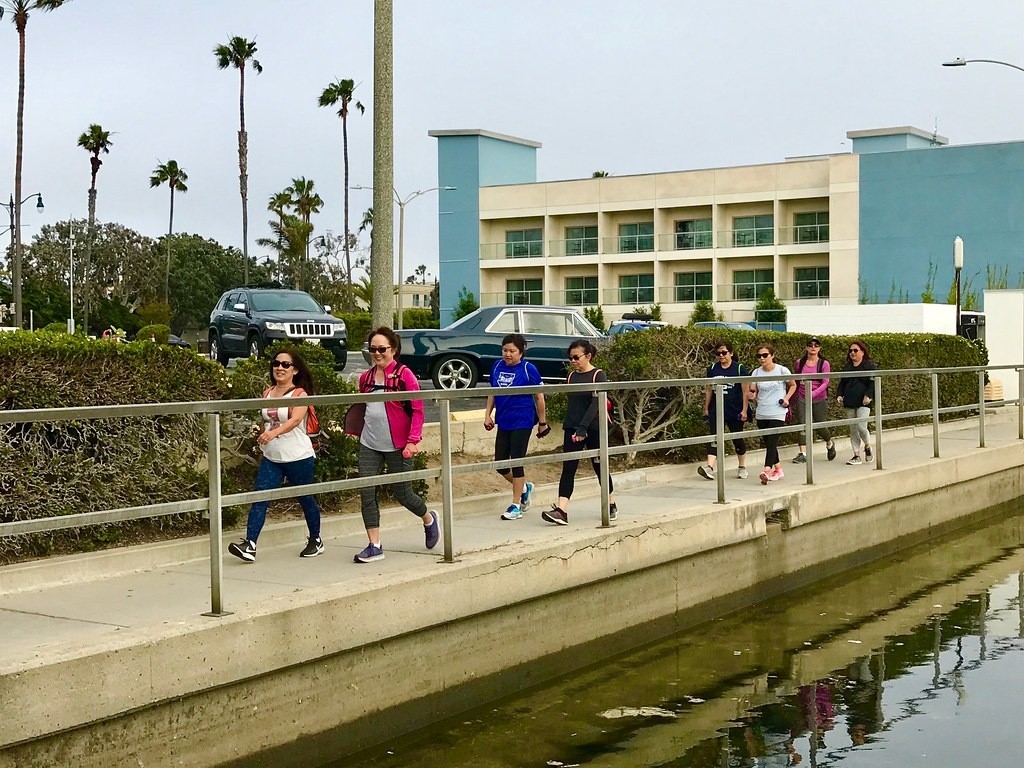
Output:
[698,340,753,480]
[793,339,836,464]
[484,334,548,519]
[226,349,324,563]
[343,326,441,563]
[836,341,878,465]
[542,339,619,526]
[747,346,796,484]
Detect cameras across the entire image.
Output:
[536,426,550,438]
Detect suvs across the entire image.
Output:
[207,285,348,371]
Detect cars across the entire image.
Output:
[605,314,671,336]
[360,304,611,389]
[693,321,756,332]
[167,334,192,349]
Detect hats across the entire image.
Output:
[808,337,821,346]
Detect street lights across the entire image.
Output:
[0,192,45,328]
[350,184,458,330]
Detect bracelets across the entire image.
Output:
[539,422,546,426]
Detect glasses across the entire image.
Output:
[849,348,858,353]
[755,353,769,359]
[271,359,294,369]
[715,350,729,356]
[568,353,586,362]
[809,343,820,347]
[368,346,392,354]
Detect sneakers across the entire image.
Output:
[500,504,523,520]
[228,538,257,562]
[736,467,749,479]
[520,482,535,513]
[542,503,569,524]
[768,468,784,481]
[827,439,836,461]
[759,471,769,485]
[423,510,440,550]
[357,542,385,563]
[846,456,863,464]
[792,453,807,464]
[610,502,618,521]
[864,445,874,463]
[698,465,715,481]
[299,536,325,557]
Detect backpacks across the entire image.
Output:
[364,364,422,418]
[262,384,322,460]
[756,366,792,423]
[568,368,615,430]
[799,357,829,395]
[710,362,754,424]
